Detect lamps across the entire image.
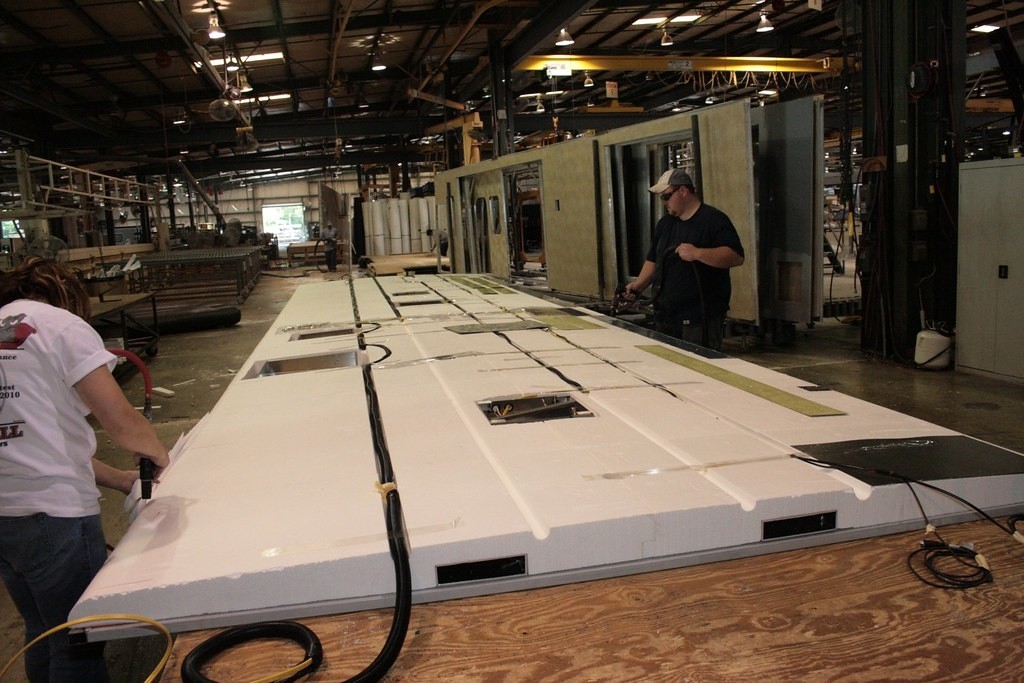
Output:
[358,95,369,108]
[371,43,386,70]
[645,71,654,81]
[756,9,774,33]
[537,100,545,112]
[554,27,574,46]
[206,11,226,39]
[705,94,713,105]
[660,24,673,46]
[239,73,253,93]
[584,71,594,87]
[225,52,239,72]
[587,96,595,107]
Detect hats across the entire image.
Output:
[326,221,332,226]
[648,168,693,194]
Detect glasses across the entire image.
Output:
[660,184,687,201]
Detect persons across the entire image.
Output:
[622,169,745,352]
[191,227,209,249]
[427,229,448,257]
[0,256,171,683]
[323,221,340,271]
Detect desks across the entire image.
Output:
[159,513,1024,683]
[84,291,159,379]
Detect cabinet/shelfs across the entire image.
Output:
[953,158,1024,387]
